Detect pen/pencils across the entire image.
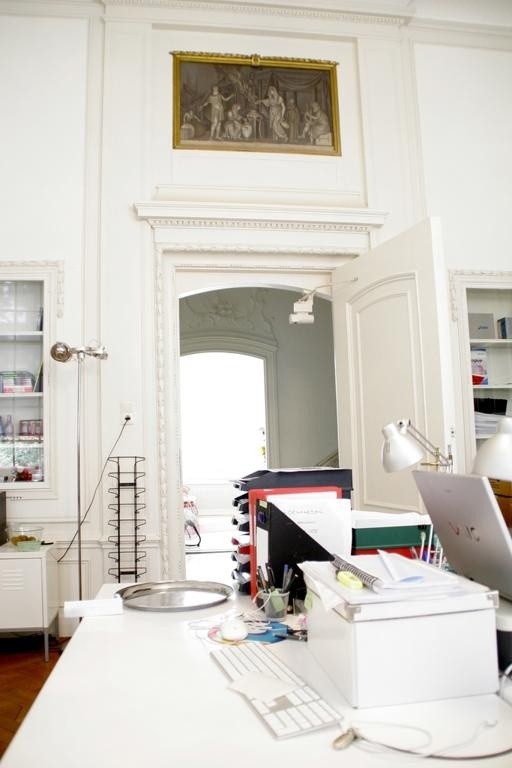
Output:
[255,565,299,592]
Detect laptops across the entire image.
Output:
[411,471,512,603]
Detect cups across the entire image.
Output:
[263,588,290,620]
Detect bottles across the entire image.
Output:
[31,462,42,483]
[0,415,43,444]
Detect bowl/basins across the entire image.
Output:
[5,525,44,546]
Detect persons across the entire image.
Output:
[181,85,331,145]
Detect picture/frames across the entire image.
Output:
[170,49,342,156]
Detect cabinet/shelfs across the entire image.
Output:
[0,268,58,493]
[446,272,512,438]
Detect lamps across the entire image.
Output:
[380,422,464,567]
[476,419,512,484]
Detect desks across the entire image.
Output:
[1,542,55,663]
[5,585,512,767]
